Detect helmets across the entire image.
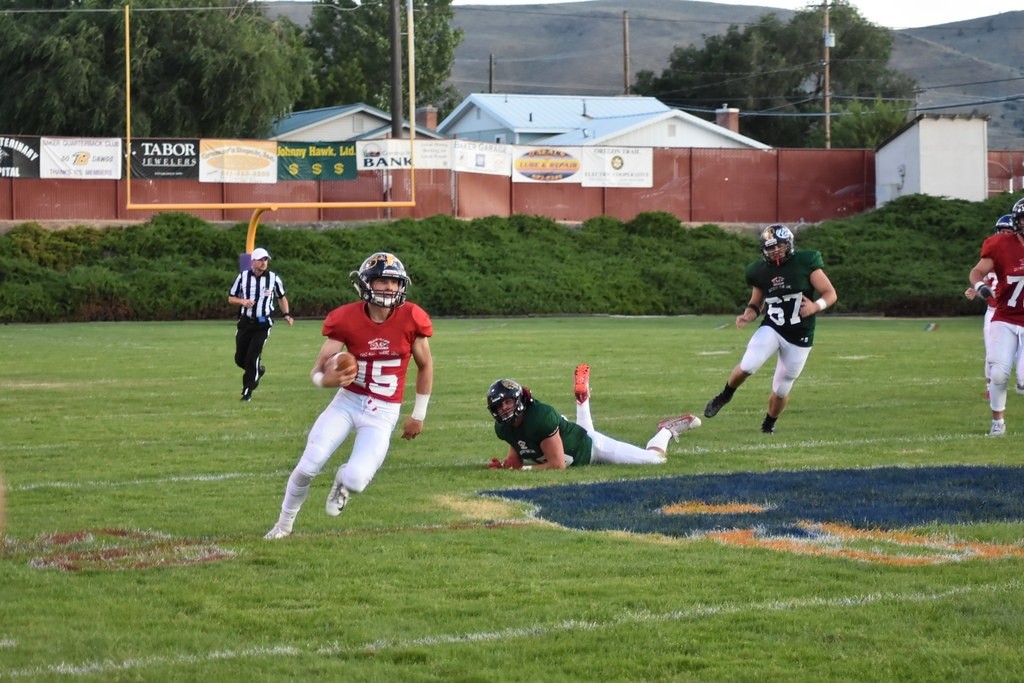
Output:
[487,379,523,425]
[996,214,1013,229]
[1011,198,1024,220]
[760,224,794,262]
[359,252,409,306]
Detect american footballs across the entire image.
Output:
[322,352,358,380]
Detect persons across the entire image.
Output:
[487,364,701,471]
[263,252,434,542]
[703,224,838,434]
[228,248,294,401]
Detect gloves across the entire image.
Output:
[977,285,991,300]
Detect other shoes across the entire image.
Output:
[704,391,731,418]
[986,390,990,400]
[252,366,265,389]
[1016,383,1024,395]
[658,414,702,443]
[989,421,1005,435]
[326,463,351,516]
[574,364,590,405]
[240,396,251,401]
[762,421,775,434]
[263,524,290,539]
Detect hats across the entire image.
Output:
[251,248,271,261]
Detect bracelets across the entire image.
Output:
[746,304,761,318]
[974,280,985,291]
[283,312,290,318]
[965,197,1024,437]
[815,298,827,311]
